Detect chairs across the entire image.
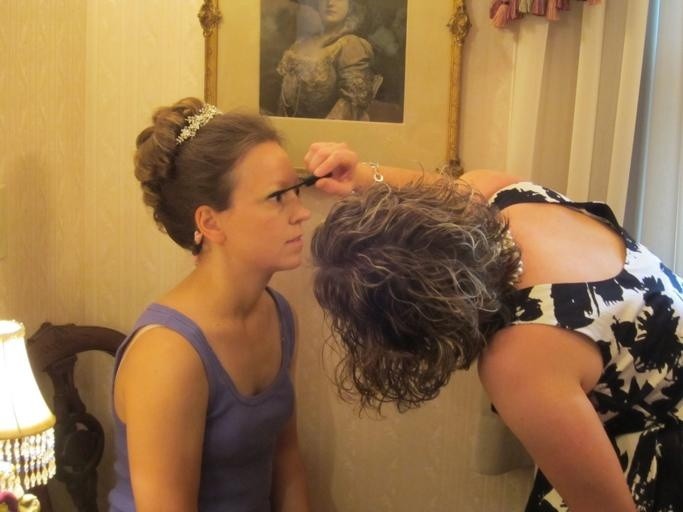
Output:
[24,320,129,512]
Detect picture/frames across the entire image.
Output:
[196,0,473,181]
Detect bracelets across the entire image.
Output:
[370,160,384,184]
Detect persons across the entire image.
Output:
[275,0,381,121]
[298,135,682,512]
[107,95,312,510]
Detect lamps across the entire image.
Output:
[0,318,58,512]
